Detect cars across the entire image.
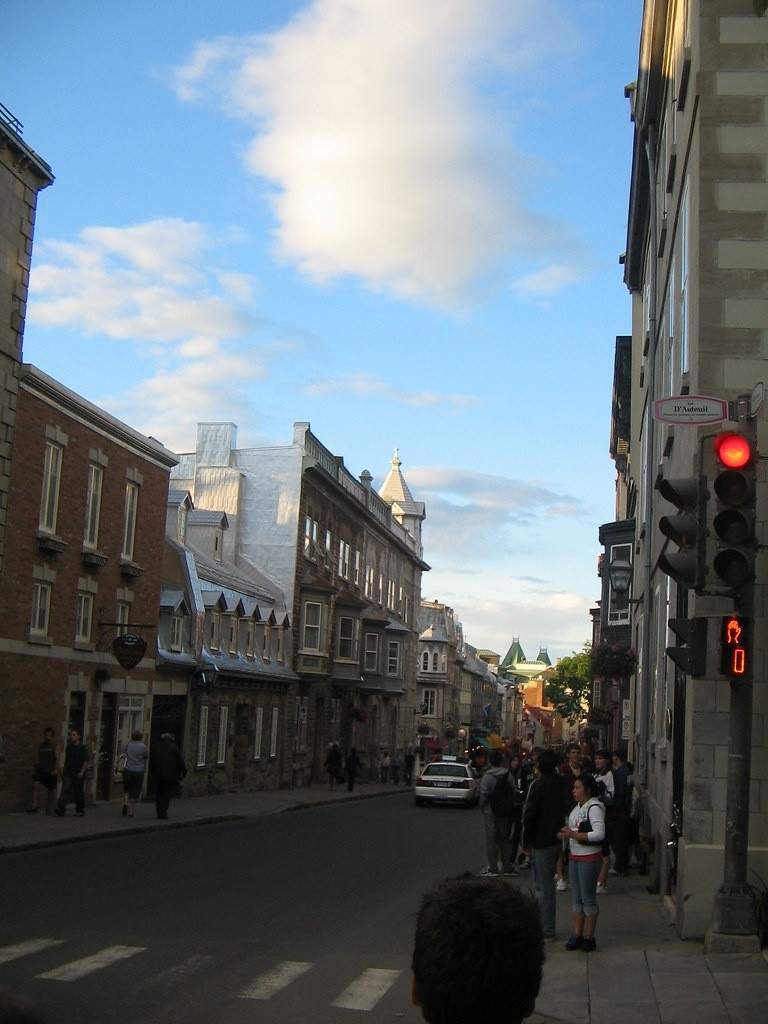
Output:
[413,755,481,808]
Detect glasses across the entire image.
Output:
[569,751,580,754]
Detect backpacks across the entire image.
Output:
[486,771,516,817]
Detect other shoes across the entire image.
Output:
[581,936,597,951]
[54,808,65,817]
[45,809,51,815]
[330,787,337,790]
[504,870,519,876]
[596,886,607,894]
[26,807,38,812]
[73,812,85,816]
[556,880,568,890]
[565,935,583,951]
[608,869,618,873]
[122,804,127,816]
[129,811,134,817]
[158,814,168,819]
[519,860,530,869]
[481,868,499,877]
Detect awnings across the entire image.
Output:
[474,734,506,749]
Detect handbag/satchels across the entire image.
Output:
[337,775,346,784]
[113,742,128,773]
[577,804,602,845]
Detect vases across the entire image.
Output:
[603,663,621,677]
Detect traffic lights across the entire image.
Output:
[663,616,706,679]
[719,614,751,681]
[710,427,759,592]
[658,477,709,590]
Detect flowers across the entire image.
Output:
[589,640,637,677]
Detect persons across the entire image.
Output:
[324,744,342,791]
[117,730,149,818]
[379,752,390,783]
[555,775,606,953]
[410,871,546,1024]
[343,748,362,793]
[520,752,576,943]
[152,730,187,820]
[53,729,90,817]
[26,727,61,816]
[404,749,415,787]
[391,750,402,785]
[474,743,644,895]
